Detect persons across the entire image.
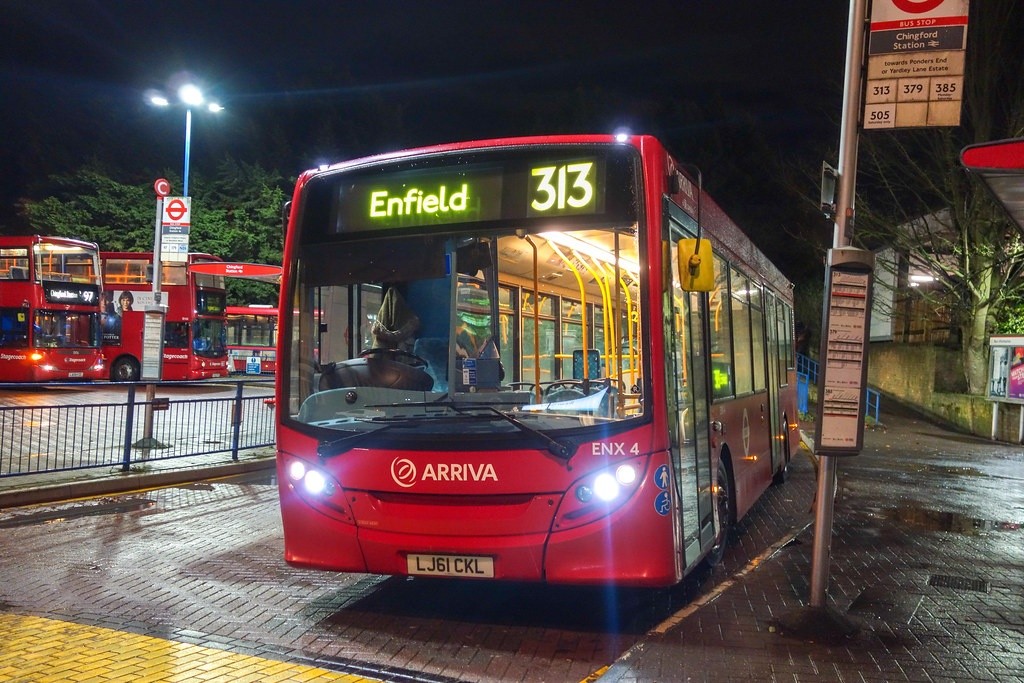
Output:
[997,360,1007,393]
[100,290,135,311]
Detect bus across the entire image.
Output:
[271,132,801,588]
[225,303,371,375]
[0,234,104,389]
[96,251,230,385]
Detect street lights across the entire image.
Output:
[142,83,223,197]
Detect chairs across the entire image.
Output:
[504,379,626,416]
